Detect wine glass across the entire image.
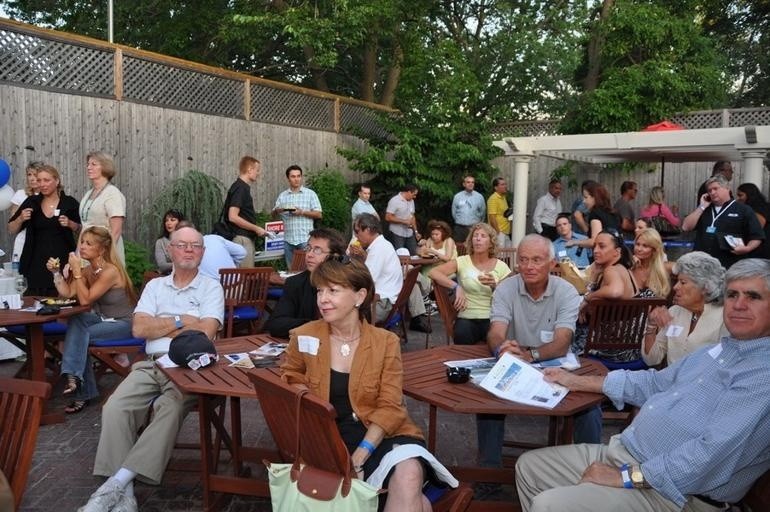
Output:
[14,277,27,311]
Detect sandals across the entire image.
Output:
[66,400,90,414]
[62,374,80,396]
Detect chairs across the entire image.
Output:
[0,241,678,511]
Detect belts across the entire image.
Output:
[695,494,726,508]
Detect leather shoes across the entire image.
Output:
[429,289,453,300]
[409,317,432,333]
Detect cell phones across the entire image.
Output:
[704,195,711,201]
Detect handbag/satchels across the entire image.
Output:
[215,220,236,240]
[262,459,385,512]
[652,214,681,237]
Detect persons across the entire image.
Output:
[0,155,769,512]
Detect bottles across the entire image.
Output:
[11,254,20,277]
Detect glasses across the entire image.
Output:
[303,245,322,253]
[326,253,355,266]
[604,226,620,238]
[172,241,203,249]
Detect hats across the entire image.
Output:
[169,331,217,369]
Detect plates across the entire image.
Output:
[42,299,78,307]
[411,257,422,260]
[282,208,296,212]
[423,256,434,259]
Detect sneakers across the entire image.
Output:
[78,477,138,512]
[473,482,501,499]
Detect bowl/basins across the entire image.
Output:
[446,367,470,385]
